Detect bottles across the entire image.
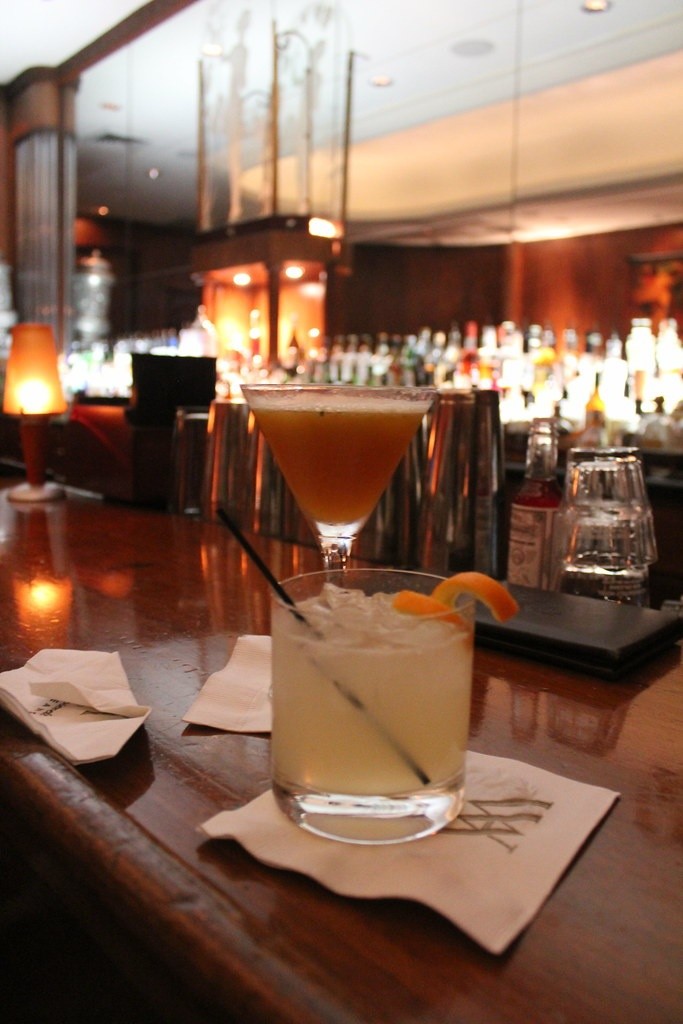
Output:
[506,418,560,594]
[188,306,683,444]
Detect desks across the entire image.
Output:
[0,469,683,1024]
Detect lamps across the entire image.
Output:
[4,323,67,504]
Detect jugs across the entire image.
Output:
[172,389,507,581]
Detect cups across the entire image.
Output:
[550,446,658,608]
[268,567,475,844]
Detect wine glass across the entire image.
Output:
[238,383,438,586]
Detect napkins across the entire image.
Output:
[0,648,152,764]
[200,751,622,955]
[183,633,273,734]
